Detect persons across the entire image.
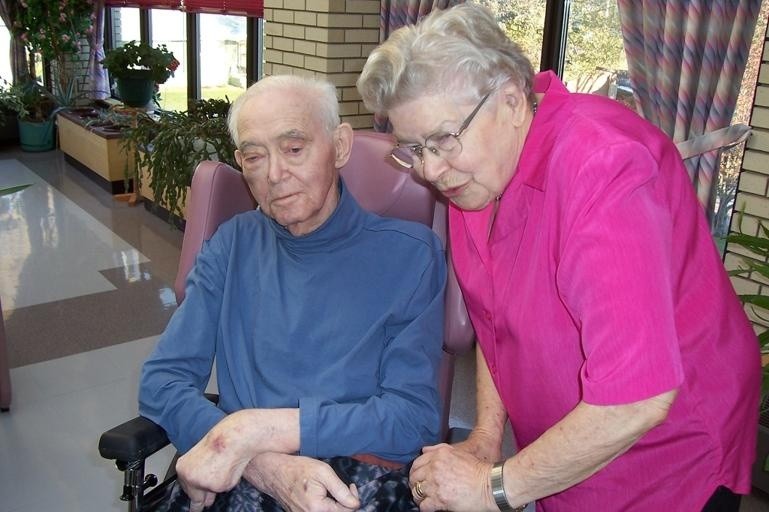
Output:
[138,75,447,512]
[357,4,762,512]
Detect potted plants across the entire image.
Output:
[125,95,241,228]
[0,75,58,152]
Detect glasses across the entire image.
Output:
[391,85,495,169]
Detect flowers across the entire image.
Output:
[10,0,178,85]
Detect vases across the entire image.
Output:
[116,76,155,107]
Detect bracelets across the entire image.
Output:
[490,460,529,512]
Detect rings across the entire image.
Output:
[414,482,424,498]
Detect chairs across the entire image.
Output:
[97,126,475,510]
